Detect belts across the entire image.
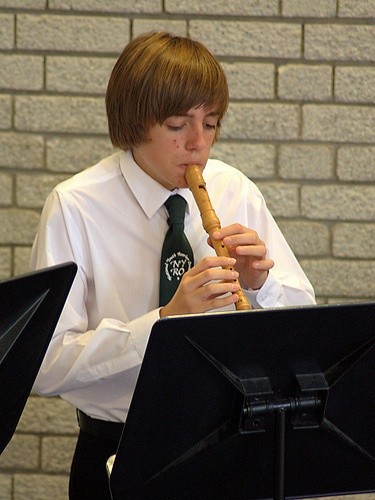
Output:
[76,411,122,440]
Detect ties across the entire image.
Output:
[158,194,194,307]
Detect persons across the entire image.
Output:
[24,28,322,500]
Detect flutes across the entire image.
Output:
[184,164,251,312]
